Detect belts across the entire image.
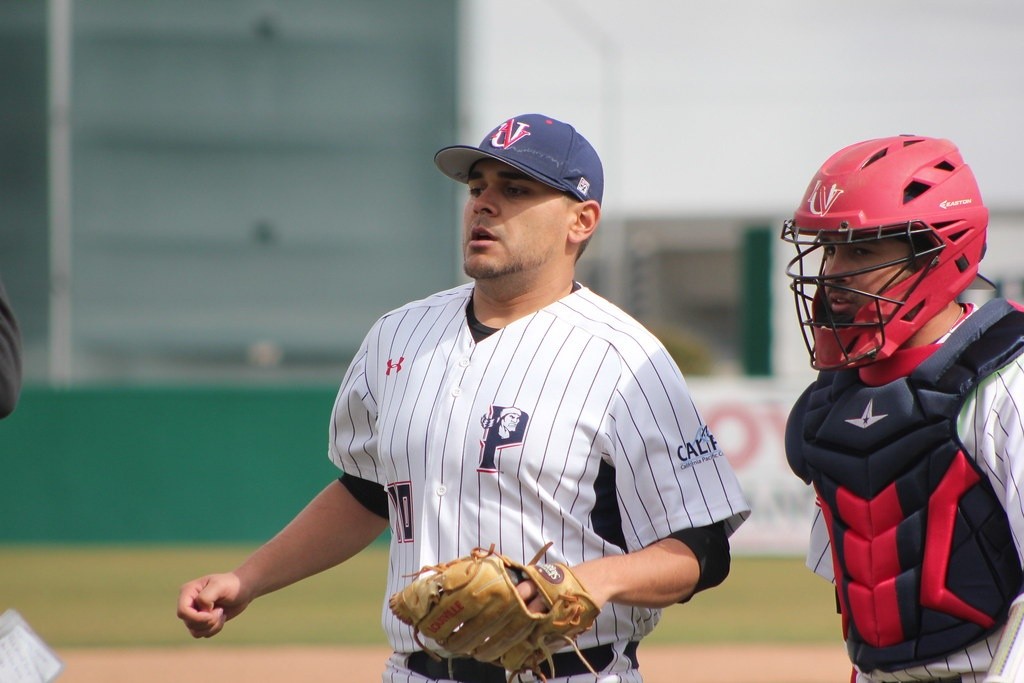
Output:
[408,641,639,683]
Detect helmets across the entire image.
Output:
[791,134,988,370]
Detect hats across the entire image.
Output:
[433,114,603,209]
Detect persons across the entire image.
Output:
[0,282,23,421]
[180,112,750,681]
[781,134,1023,683]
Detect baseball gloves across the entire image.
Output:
[386,539,604,683]
